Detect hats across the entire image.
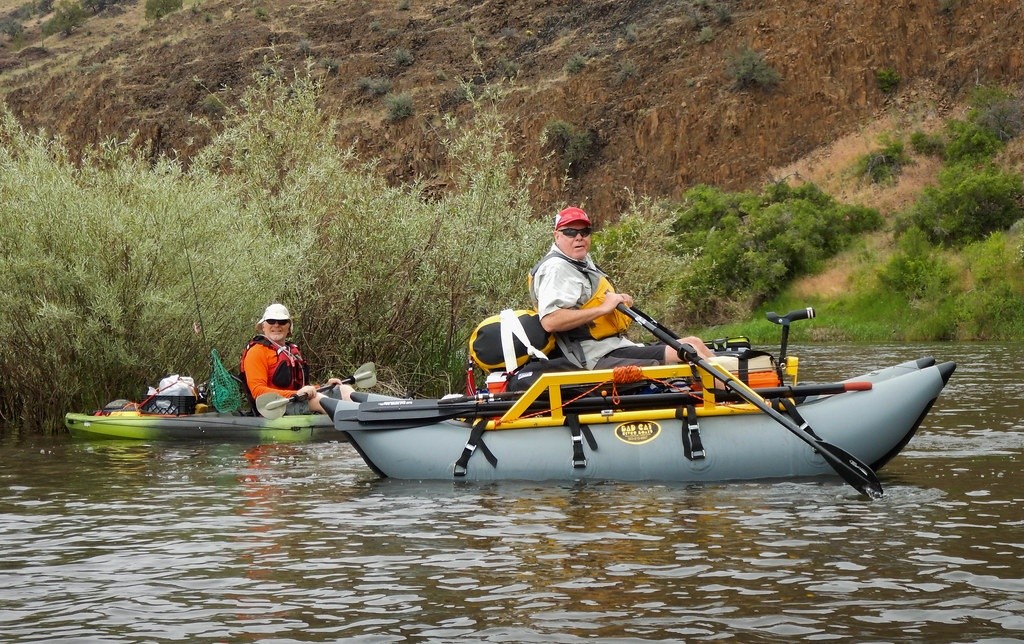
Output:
[255,304,293,337]
[555,207,592,230]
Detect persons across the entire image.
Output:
[241,303,357,415]
[528,207,717,371]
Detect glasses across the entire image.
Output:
[558,227,591,238]
[266,319,290,325]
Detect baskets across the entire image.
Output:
[140,394,196,416]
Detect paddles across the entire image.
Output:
[256,362,377,420]
[628,306,681,340]
[334,381,872,431]
[616,303,884,500]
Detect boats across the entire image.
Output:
[63,405,348,447]
[319,308,960,489]
